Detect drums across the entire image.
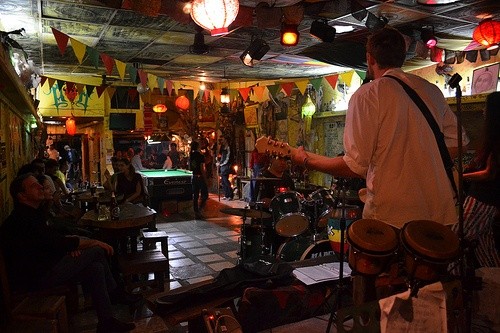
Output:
[238,223,271,258]
[306,187,342,229]
[345,217,398,276]
[277,234,336,262]
[399,216,459,282]
[271,191,310,238]
[329,205,361,255]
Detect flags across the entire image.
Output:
[350,1,491,64]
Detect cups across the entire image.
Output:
[98,205,108,221]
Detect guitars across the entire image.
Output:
[254,135,460,216]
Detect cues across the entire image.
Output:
[217,154,220,201]
[171,153,185,173]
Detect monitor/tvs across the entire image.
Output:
[111,86,140,109]
[109,113,136,130]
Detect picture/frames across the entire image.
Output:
[470,62,500,95]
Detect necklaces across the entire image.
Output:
[303,157,314,171]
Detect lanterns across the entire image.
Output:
[472,17,500,51]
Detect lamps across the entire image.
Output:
[310,19,336,44]
[219,65,231,116]
[279,15,299,45]
[241,32,270,68]
[134,63,149,94]
[421,24,437,49]
[189,28,209,54]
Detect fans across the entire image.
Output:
[269,92,284,109]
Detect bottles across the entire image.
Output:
[110,191,120,221]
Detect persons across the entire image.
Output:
[288,26,472,272]
[250,154,301,258]
[104,141,181,208]
[448,89,500,277]
[188,142,209,221]
[215,137,235,201]
[0,143,136,333]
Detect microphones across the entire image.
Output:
[362,73,371,85]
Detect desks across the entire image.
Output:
[81,203,156,254]
[71,186,104,197]
[137,169,193,186]
[79,190,112,212]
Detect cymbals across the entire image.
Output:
[241,177,282,181]
[220,208,272,219]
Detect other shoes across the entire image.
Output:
[96,317,136,333]
[109,291,143,306]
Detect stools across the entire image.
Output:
[143,232,168,261]
[7,283,79,333]
[132,250,169,293]
